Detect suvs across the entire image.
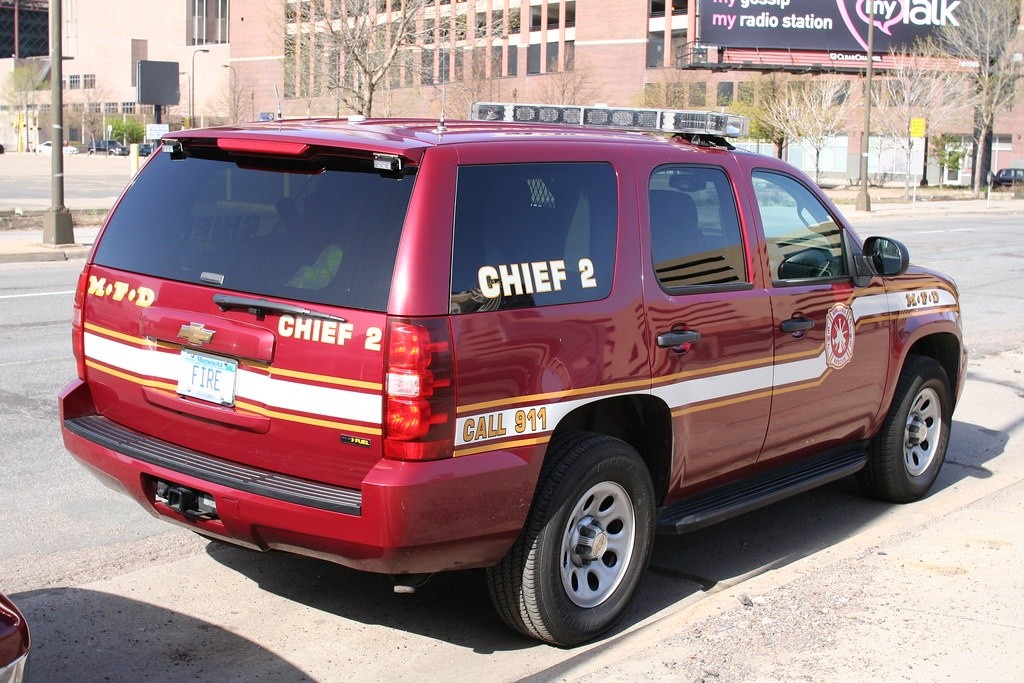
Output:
[57,102,969,650]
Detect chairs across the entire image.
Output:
[649,188,697,261]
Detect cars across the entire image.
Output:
[993,168,1024,186]
[139,144,152,156]
[88,140,130,156]
[36,141,79,155]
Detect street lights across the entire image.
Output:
[192,49,210,128]
[223,65,237,79]
[181,72,191,115]
